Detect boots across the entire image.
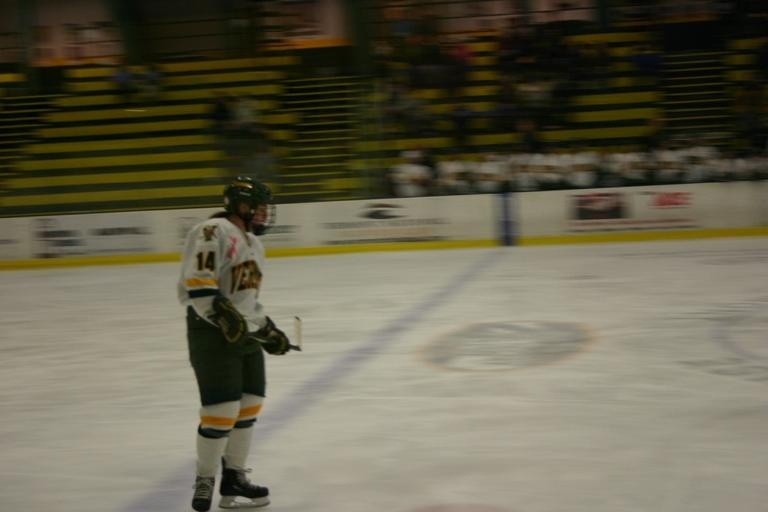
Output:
[191,475,215,511]
[219,456,269,498]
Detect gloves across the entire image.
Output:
[253,316,289,355]
[206,295,246,346]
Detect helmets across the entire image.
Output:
[222,176,277,236]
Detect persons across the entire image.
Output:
[205,90,236,133]
[233,90,262,137]
[109,58,140,96]
[174,176,293,512]
[141,55,170,89]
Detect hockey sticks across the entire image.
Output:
[244,317,302,351]
